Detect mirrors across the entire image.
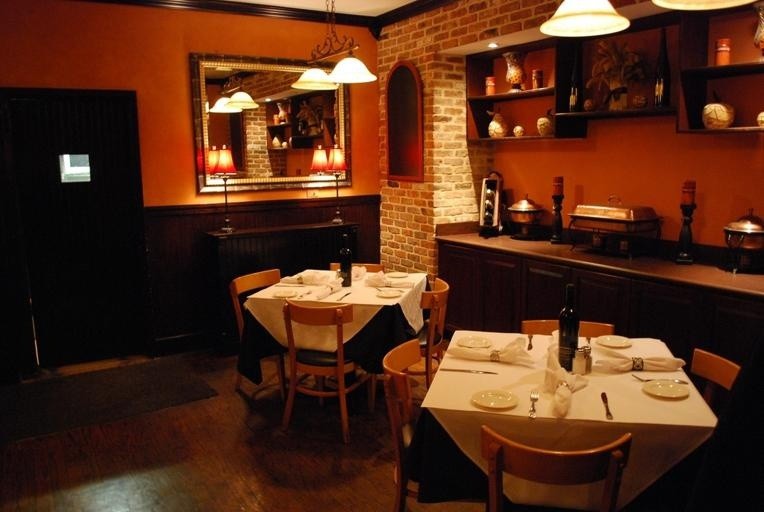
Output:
[189,51,352,194]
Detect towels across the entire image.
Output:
[317,278,344,299]
[548,328,560,349]
[596,346,687,373]
[365,271,415,289]
[280,273,330,285]
[351,266,367,280]
[544,353,589,417]
[447,337,536,369]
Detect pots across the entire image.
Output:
[507,193,545,240]
[722,207,764,274]
[567,194,664,259]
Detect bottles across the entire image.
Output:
[654,29,669,110]
[532,69,541,88]
[568,43,581,112]
[339,235,352,287]
[559,284,579,371]
[486,77,496,95]
[715,38,730,66]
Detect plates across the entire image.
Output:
[642,380,688,398]
[374,288,402,298]
[471,389,518,409]
[273,290,296,297]
[595,335,632,348]
[385,271,409,278]
[456,335,492,348]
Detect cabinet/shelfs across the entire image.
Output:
[291,90,337,148]
[675,2,764,133]
[466,37,588,141]
[554,10,680,118]
[209,221,360,357]
[436,235,764,512]
[265,94,289,151]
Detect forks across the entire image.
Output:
[631,373,689,384]
[527,389,539,419]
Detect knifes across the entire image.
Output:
[337,291,352,302]
[600,392,613,420]
[527,334,532,350]
[439,368,498,375]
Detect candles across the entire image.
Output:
[551,173,563,193]
[681,176,695,207]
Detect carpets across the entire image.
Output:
[3,356,217,444]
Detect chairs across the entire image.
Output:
[282,301,376,445]
[382,338,487,511]
[403,278,449,388]
[692,347,740,407]
[330,263,384,273]
[523,320,612,338]
[230,270,314,398]
[478,424,630,512]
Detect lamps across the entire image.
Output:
[327,145,349,223]
[214,145,237,234]
[310,144,326,176]
[539,0,630,37]
[289,63,339,90]
[208,144,220,178]
[291,1,378,91]
[209,69,260,114]
